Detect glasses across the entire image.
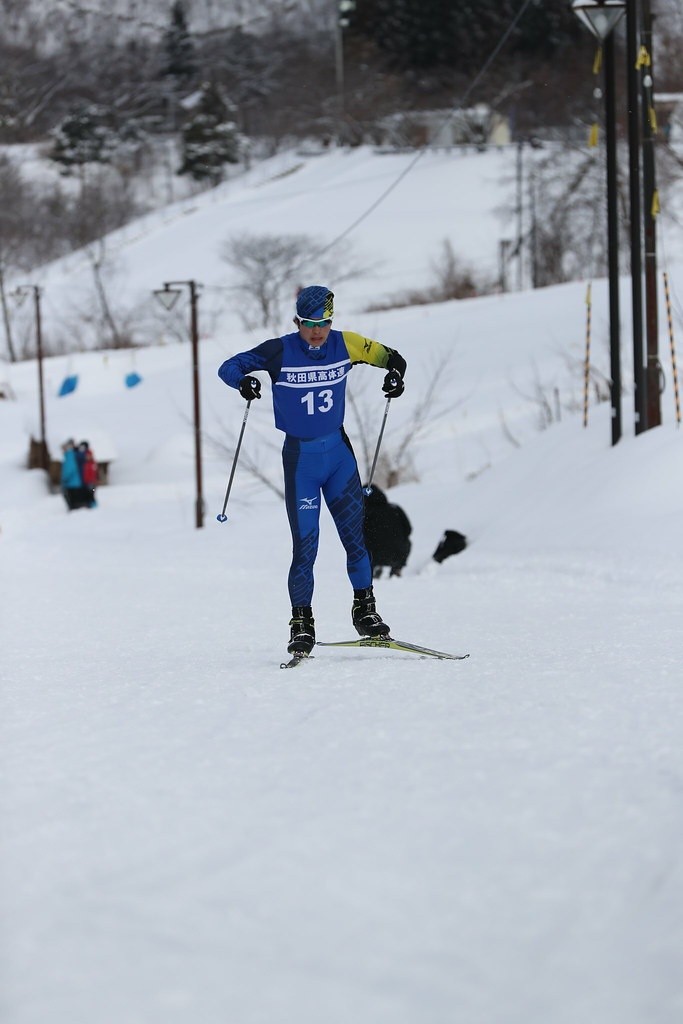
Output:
[297,315,333,328]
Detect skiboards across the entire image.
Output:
[279,633,471,667]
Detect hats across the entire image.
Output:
[297,287,334,320]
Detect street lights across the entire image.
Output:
[11,283,47,456]
[154,280,204,524]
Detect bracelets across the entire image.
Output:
[392,368,401,377]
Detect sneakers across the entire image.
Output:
[287,607,315,653]
[353,588,390,636]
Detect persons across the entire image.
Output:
[363,483,413,580]
[58,439,98,510]
[218,286,408,658]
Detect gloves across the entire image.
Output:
[382,372,404,398]
[239,377,261,400]
